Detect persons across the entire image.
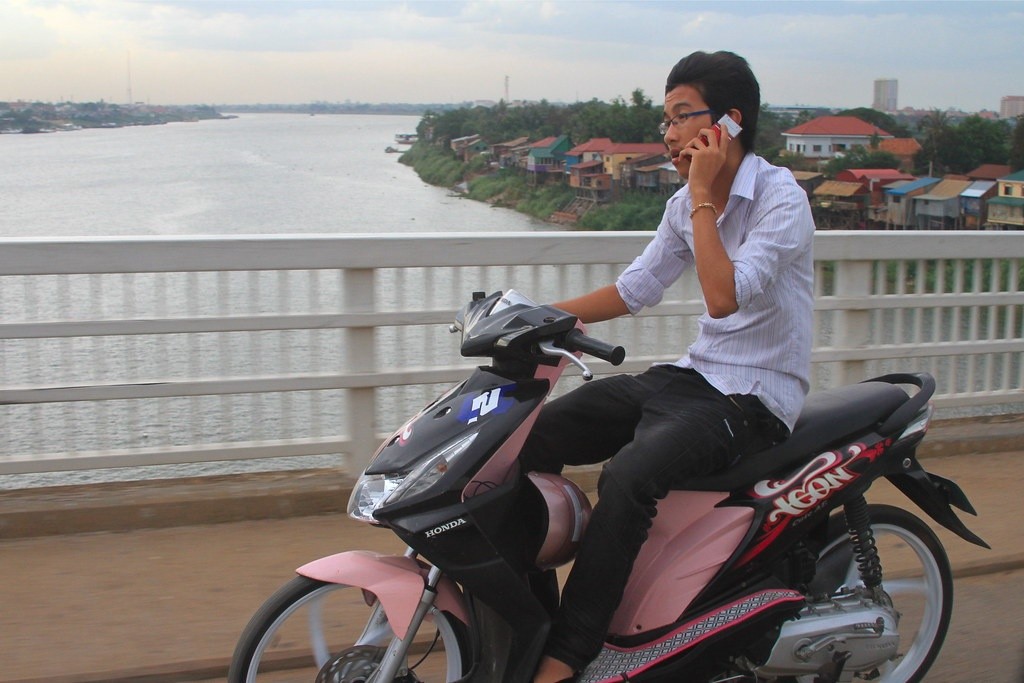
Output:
[520,50,818,683]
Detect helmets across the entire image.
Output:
[515,469,593,570]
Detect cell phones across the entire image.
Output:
[684,114,743,163]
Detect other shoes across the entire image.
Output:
[529,648,584,683]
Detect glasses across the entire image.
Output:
[659,109,715,134]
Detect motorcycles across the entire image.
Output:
[224,285,994,683]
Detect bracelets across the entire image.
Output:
[691,202,717,217]
